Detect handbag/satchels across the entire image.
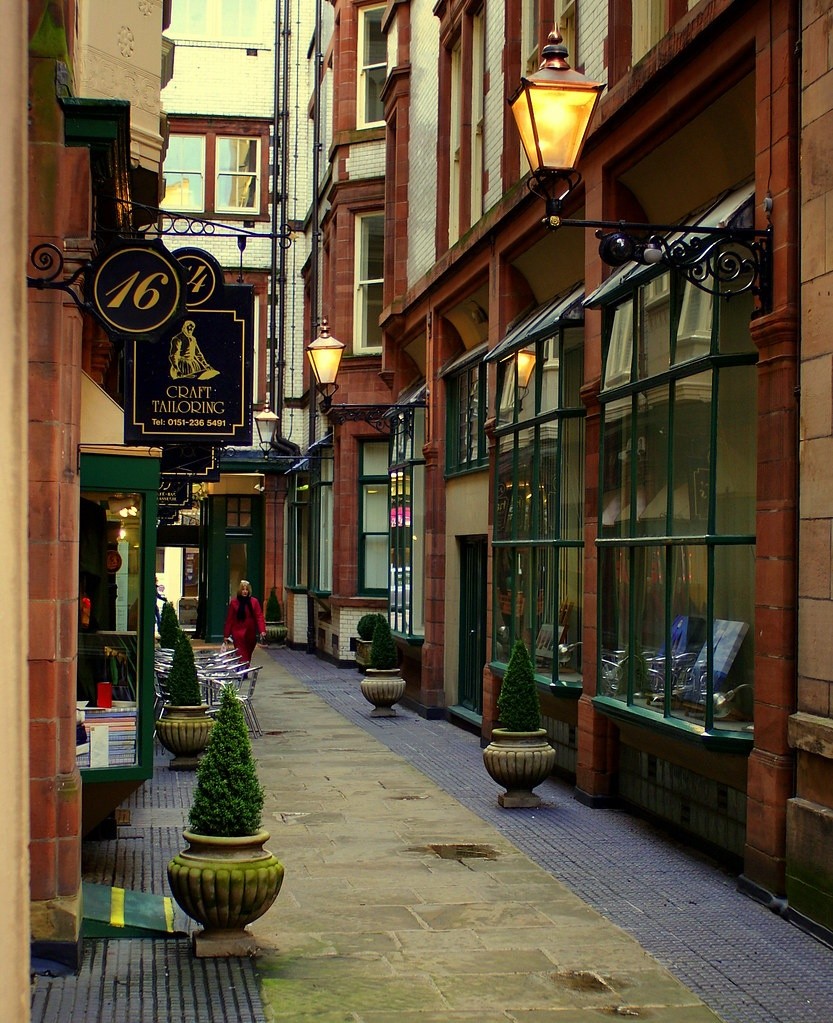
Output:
[220,642,227,654]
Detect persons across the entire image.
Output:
[223,580,267,679]
[155,577,168,632]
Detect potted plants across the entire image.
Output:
[263,591,289,650]
[355,612,384,671]
[359,615,405,718]
[170,683,283,959]
[486,640,553,808]
[156,634,219,771]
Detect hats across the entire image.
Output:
[237,579,252,597]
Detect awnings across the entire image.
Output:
[580,181,755,310]
[308,433,333,451]
[285,459,308,474]
[437,337,488,380]
[384,380,427,417]
[482,283,586,362]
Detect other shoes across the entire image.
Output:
[244,673,248,678]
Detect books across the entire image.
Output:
[683,620,750,704]
[651,614,704,690]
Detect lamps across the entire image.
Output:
[503,31,779,323]
[306,319,429,438]
